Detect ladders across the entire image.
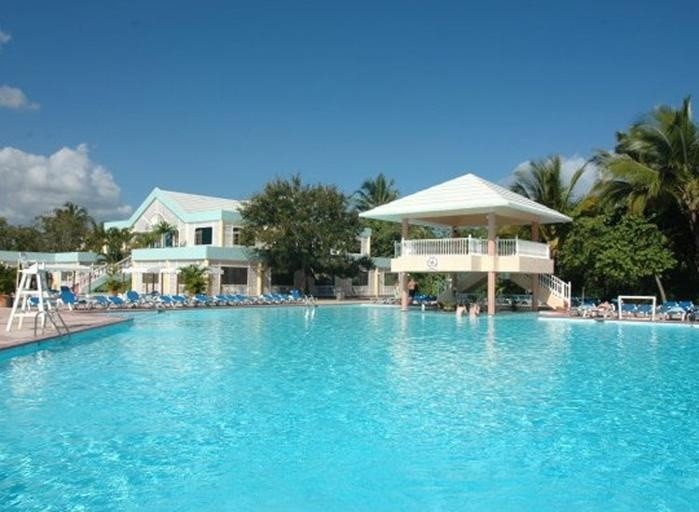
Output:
[7,264,51,332]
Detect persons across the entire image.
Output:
[452,229,460,253]
[407,277,416,304]
[455,303,468,317]
[596,300,610,311]
[468,303,480,317]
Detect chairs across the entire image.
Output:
[370,293,439,306]
[15,285,308,311]
[577,301,699,322]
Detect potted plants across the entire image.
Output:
[103,267,132,295]
[0,264,19,307]
[176,263,215,297]
